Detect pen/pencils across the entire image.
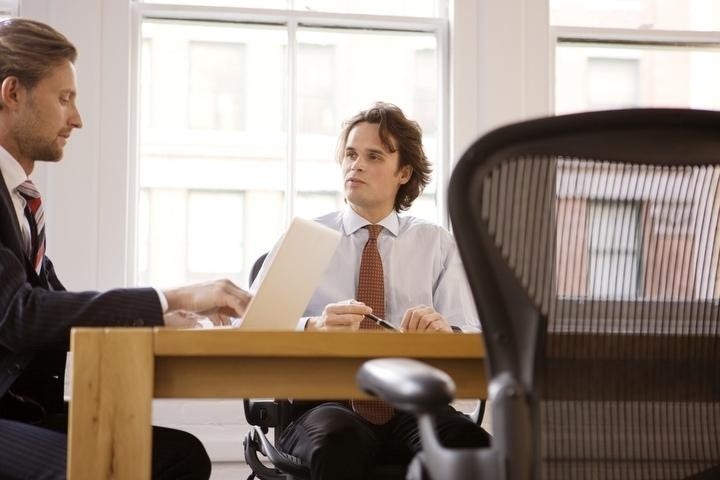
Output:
[348,300,395,330]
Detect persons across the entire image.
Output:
[229,102,483,480]
[0,20,251,479]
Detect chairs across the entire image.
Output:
[243,254,485,480]
[358,108,720,480]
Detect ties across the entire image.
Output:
[16,180,46,276]
[351,225,395,424]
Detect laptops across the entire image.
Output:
[181,216,343,330]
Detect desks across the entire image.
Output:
[65,327,720,480]
